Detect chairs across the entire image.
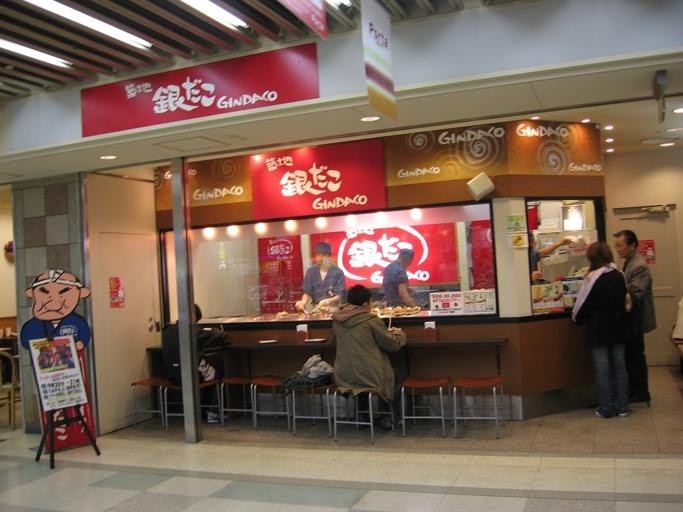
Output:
[0,349,20,431]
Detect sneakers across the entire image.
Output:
[617,408,631,417]
[207,412,229,424]
[592,409,607,416]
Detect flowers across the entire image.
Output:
[4,240,13,252]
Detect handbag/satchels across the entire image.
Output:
[608,311,641,344]
[282,370,333,389]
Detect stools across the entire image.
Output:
[193,380,222,421]
[220,376,252,427]
[451,372,506,440]
[129,377,165,430]
[291,381,335,437]
[163,381,195,431]
[400,375,451,437]
[335,382,395,441]
[249,375,287,428]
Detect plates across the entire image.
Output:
[259,339,278,343]
[304,338,326,342]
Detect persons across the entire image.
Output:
[528,230,571,285]
[38,345,74,369]
[612,229,657,407]
[327,286,407,429]
[383,249,415,307]
[162,305,228,424]
[572,242,634,419]
[295,241,346,310]
[20,269,92,352]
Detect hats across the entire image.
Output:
[317,243,331,255]
[399,249,413,259]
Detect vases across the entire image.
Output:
[5,252,14,263]
